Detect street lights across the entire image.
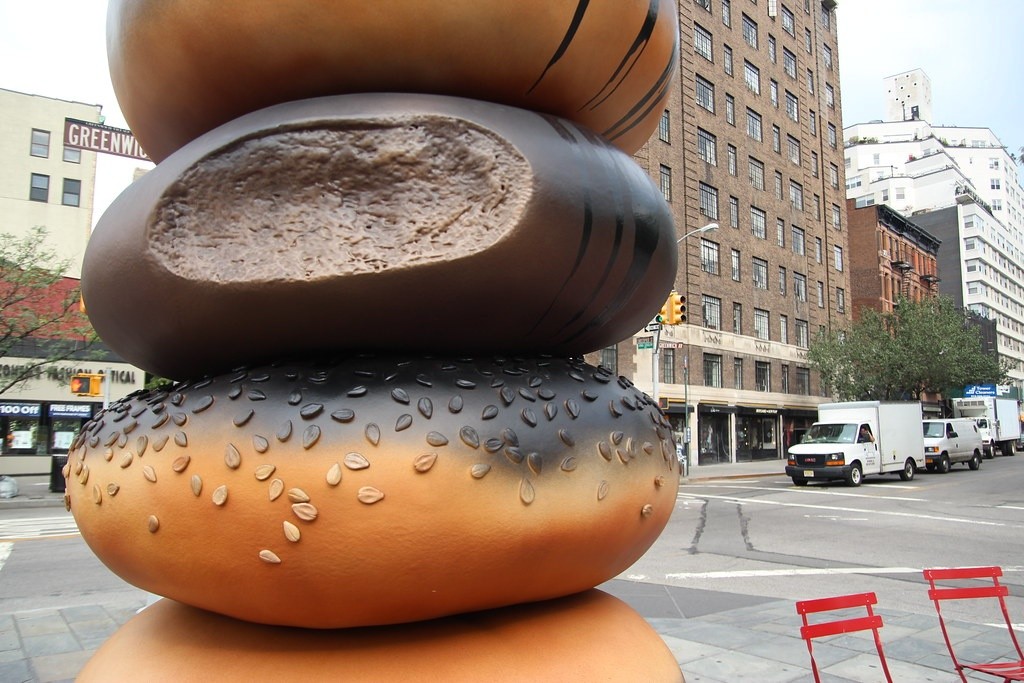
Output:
[651,222,720,406]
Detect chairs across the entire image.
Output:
[923,566,1024,683]
[795,592,893,683]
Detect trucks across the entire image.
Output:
[784,395,1024,487]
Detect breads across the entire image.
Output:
[60,0,693,683]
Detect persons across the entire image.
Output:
[858,427,875,443]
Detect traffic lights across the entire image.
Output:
[653,289,686,327]
